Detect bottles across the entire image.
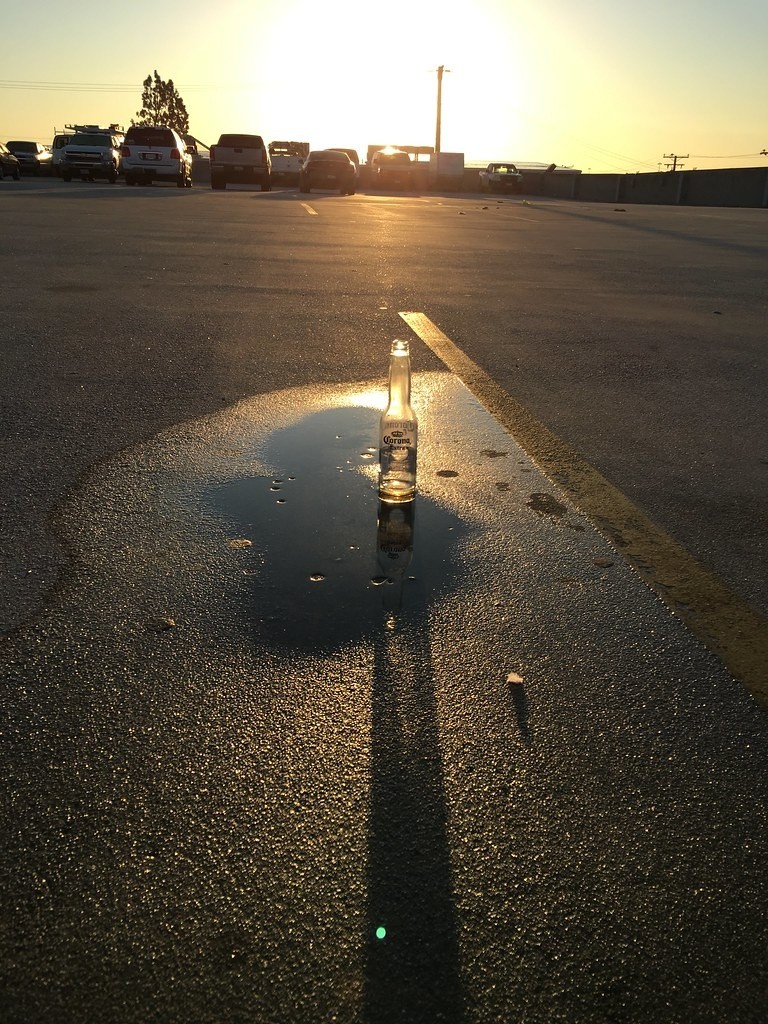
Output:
[379,340,419,503]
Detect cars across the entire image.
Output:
[299,150,359,195]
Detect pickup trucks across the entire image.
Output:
[210,133,275,192]
[479,162,526,194]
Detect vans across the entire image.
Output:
[0,124,310,188]
[371,150,412,192]
